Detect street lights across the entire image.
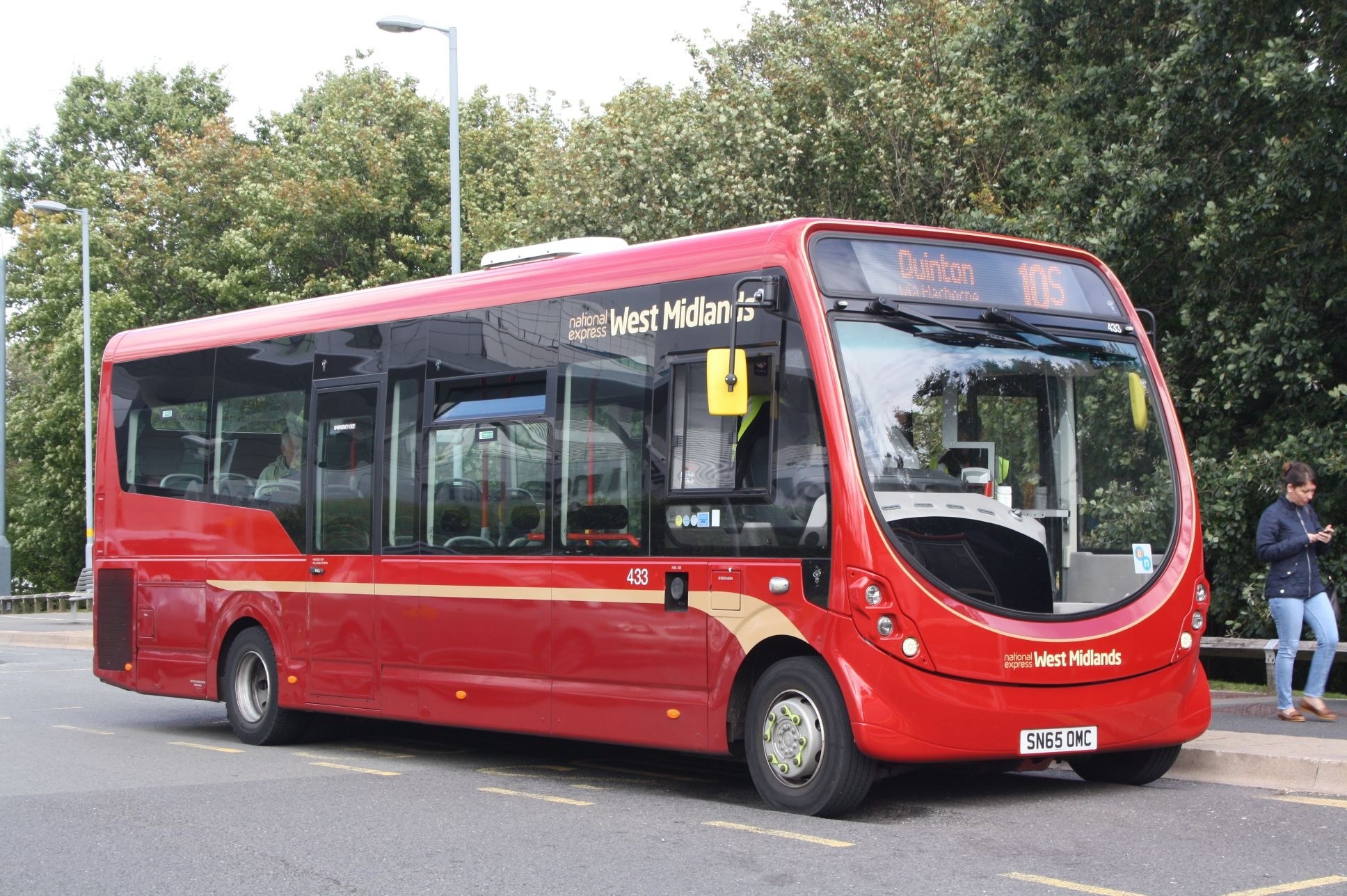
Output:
[375,14,461,483]
[32,200,93,610]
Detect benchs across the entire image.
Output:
[69,566,95,611]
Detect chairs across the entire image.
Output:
[738,404,818,504]
[158,472,629,556]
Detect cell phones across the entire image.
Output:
[1323,530,1330,534]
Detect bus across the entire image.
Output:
[93,217,1212,818]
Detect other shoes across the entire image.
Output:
[1300,697,1337,720]
[1277,707,1306,722]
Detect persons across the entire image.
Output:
[254,423,315,499]
[938,411,1025,509]
[1257,461,1338,721]
